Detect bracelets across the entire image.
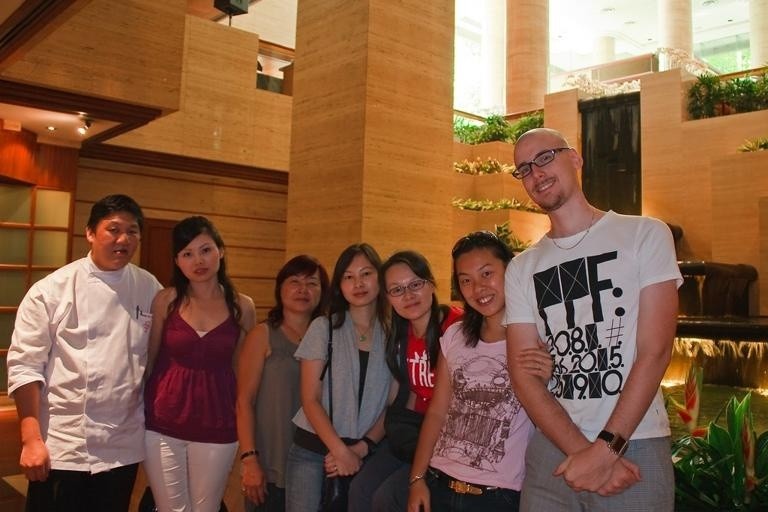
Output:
[408,471,426,487]
[239,448,259,461]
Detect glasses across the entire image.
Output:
[452,230,499,256]
[512,147,568,180]
[385,279,429,297]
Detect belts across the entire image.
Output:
[428,467,504,495]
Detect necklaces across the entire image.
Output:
[550,205,595,250]
[414,325,427,338]
[353,314,375,342]
[286,323,304,342]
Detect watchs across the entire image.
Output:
[597,428,630,457]
[361,435,378,456]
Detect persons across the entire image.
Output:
[139,215,259,512]
[233,252,331,511]
[502,124,686,512]
[6,191,166,512]
[404,228,555,512]
[280,243,405,511]
[346,248,464,512]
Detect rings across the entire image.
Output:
[334,465,337,471]
[240,488,247,492]
